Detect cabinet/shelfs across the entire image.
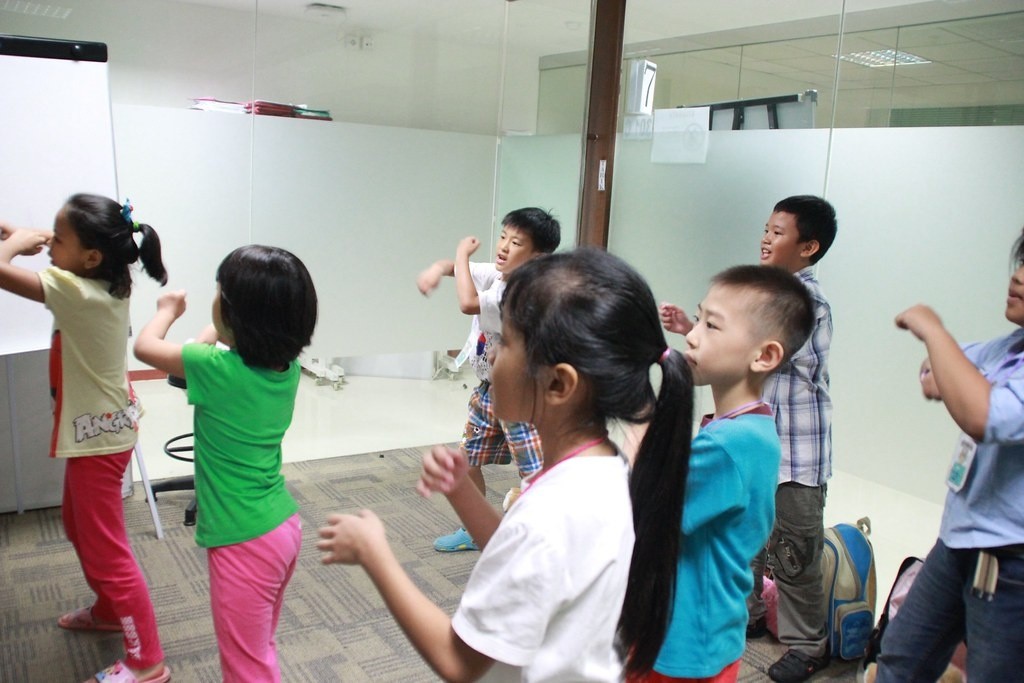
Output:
[0,336,134,513]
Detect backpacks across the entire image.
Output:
[863,557,926,669]
[821,516,877,665]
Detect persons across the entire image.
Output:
[315,248,694,683]
[416,208,561,553]
[0,193,171,683]
[133,245,317,683]
[873,230,1024,683]
[621,195,837,683]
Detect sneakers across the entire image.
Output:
[747,616,768,640]
[768,649,831,683]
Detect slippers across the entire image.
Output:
[82,660,172,683]
[432,528,481,551]
[58,606,122,631]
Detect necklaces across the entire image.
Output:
[701,397,764,429]
[520,434,604,495]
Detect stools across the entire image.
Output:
[145,374,198,526]
